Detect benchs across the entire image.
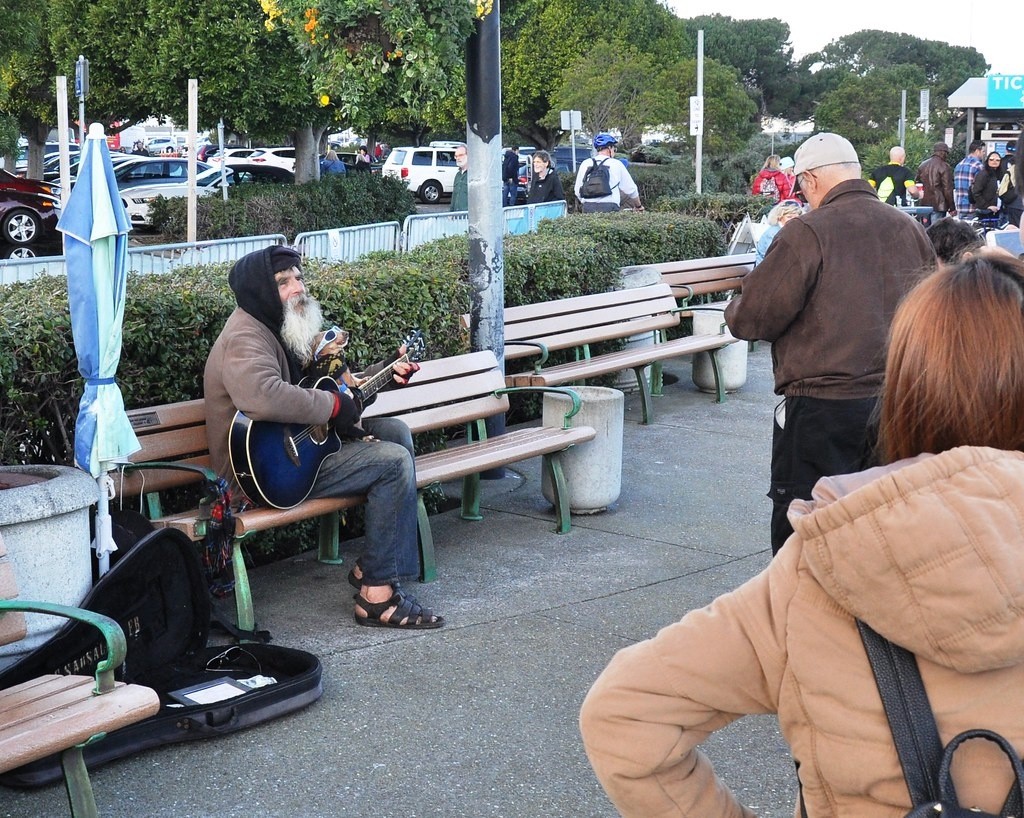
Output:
[461,282,741,426]
[617,252,758,399]
[0,530,160,818]
[107,349,597,642]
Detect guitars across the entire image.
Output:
[228,328,425,512]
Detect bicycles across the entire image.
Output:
[958,208,1011,242]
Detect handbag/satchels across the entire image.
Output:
[1000,171,1018,205]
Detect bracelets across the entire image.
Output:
[635,205,641,208]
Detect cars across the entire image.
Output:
[0,136,385,262]
[428,141,630,204]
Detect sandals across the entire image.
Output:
[349,559,415,604]
[352,587,447,629]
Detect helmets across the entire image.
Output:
[593,133,618,148]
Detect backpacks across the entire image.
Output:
[967,169,991,204]
[580,157,621,198]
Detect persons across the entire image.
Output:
[577,250,1024,818]
[374,144,381,157]
[724,131,939,558]
[320,150,346,176]
[927,217,979,266]
[756,199,804,267]
[867,146,920,206]
[954,128,1024,246]
[752,154,803,206]
[573,133,644,213]
[502,145,519,206]
[915,142,957,225]
[355,146,370,163]
[527,150,565,204]
[450,144,468,219]
[132,142,149,156]
[203,245,444,628]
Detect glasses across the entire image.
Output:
[1008,147,1018,152]
[454,152,467,160]
[989,156,1001,162]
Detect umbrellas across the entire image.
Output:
[54,122,143,580]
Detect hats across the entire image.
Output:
[789,131,860,199]
[272,247,301,271]
[780,157,795,170]
[934,142,949,153]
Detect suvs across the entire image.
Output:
[381,145,460,205]
